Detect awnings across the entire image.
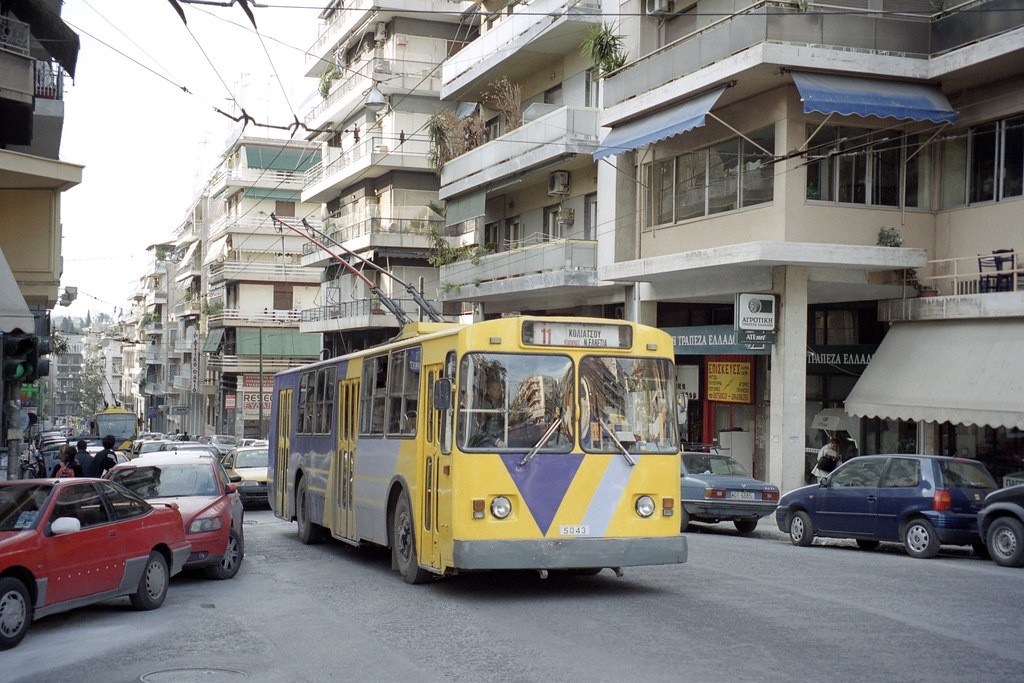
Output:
[842,320,1024,431]
[791,70,956,125]
[9,0,80,86]
[0,250,35,333]
[811,408,859,447]
[593,87,726,162]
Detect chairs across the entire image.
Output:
[992,249,1014,291]
[978,254,996,292]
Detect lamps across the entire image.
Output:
[65,285,78,300]
[59,294,67,300]
[56,301,71,307]
[364,85,386,112]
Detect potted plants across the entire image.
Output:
[372,280,384,315]
[877,227,938,296]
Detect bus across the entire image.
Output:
[268,314,687,585]
[90,407,141,450]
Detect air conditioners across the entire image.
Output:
[547,172,569,194]
[645,0,668,15]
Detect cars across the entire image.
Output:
[976,482,1024,567]
[0,479,192,652]
[776,455,1000,558]
[219,441,268,505]
[33,428,267,580]
[679,452,779,537]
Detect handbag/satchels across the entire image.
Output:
[817,456,839,473]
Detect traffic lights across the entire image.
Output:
[1,329,49,387]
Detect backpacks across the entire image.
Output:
[55,462,74,478]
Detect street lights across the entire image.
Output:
[63,390,70,425]
[53,393,58,419]
[246,320,262,438]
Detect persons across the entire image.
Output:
[51,435,118,498]
[475,380,505,449]
[811,440,857,482]
[181,432,189,441]
